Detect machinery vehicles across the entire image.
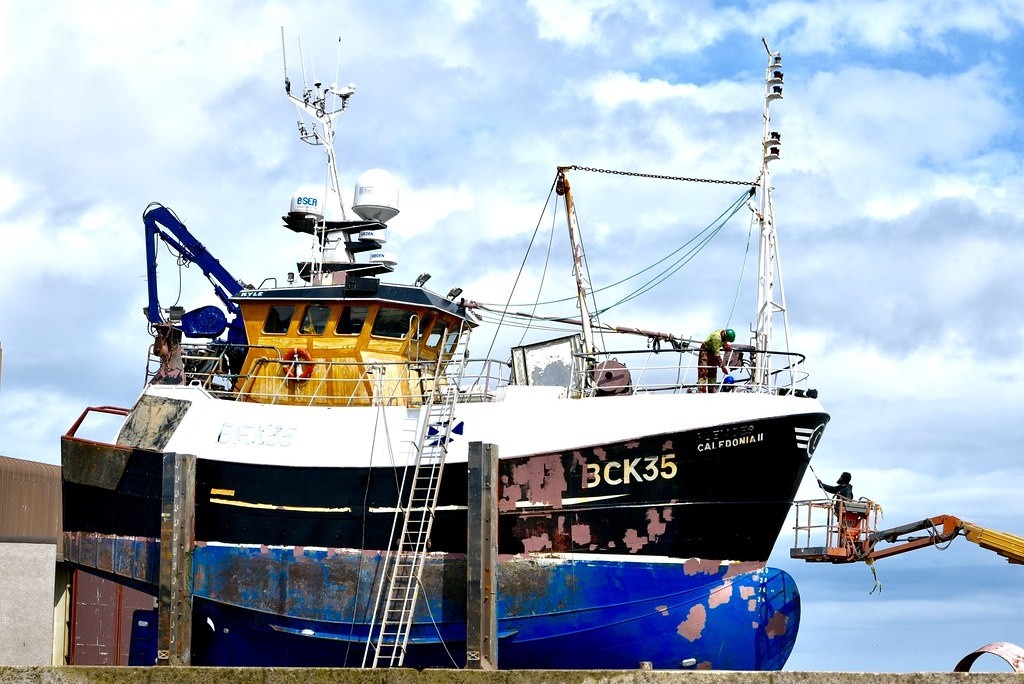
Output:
[138,201,258,387]
[790,495,1024,569]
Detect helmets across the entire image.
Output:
[724,329,735,342]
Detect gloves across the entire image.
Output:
[722,367,728,374]
[723,343,732,351]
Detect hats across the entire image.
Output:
[837,472,851,484]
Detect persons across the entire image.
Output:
[818,472,853,521]
[698,329,735,392]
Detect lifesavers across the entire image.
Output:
[283,346,314,380]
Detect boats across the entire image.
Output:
[51,20,839,668]
